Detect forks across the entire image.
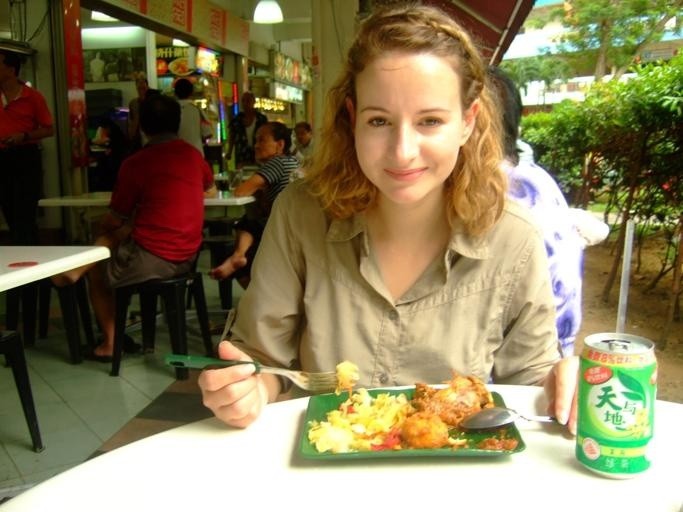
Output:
[163,352,353,392]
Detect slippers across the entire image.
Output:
[82,342,112,363]
[190,319,231,337]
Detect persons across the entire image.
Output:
[484,62,584,358]
[47,87,216,363]
[172,79,212,158]
[104,53,120,81]
[128,69,150,140]
[88,50,104,81]
[293,121,316,160]
[0,51,56,240]
[191,0,584,438]
[210,120,303,289]
[224,88,269,169]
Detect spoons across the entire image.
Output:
[459,405,568,429]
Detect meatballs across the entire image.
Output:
[404,413,449,449]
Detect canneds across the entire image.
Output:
[576,331,657,481]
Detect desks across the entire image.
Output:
[0,245,112,453]
[37,192,256,352]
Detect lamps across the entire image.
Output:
[252,0,283,25]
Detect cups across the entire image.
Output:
[228,170,243,191]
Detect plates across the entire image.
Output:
[297,390,526,458]
[167,58,195,77]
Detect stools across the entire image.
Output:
[109,271,214,380]
[5,277,98,365]
